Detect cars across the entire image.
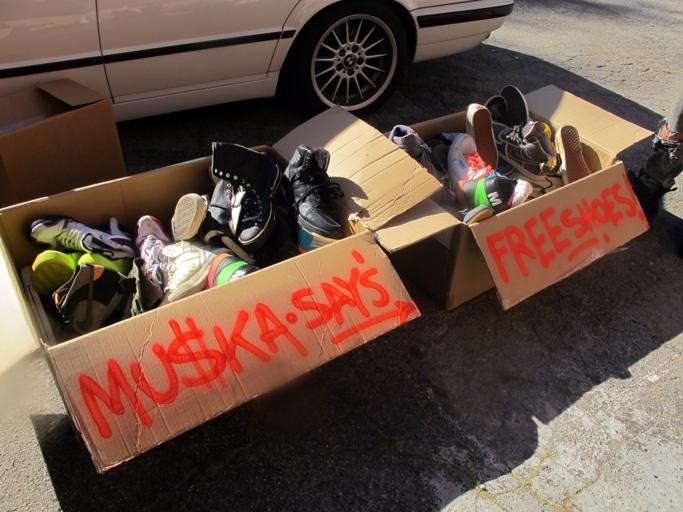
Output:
[1,0,514,124]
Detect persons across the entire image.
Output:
[627,92,682,218]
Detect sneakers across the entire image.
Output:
[25,142,344,342]
[386,84,591,225]
[626,168,677,216]
